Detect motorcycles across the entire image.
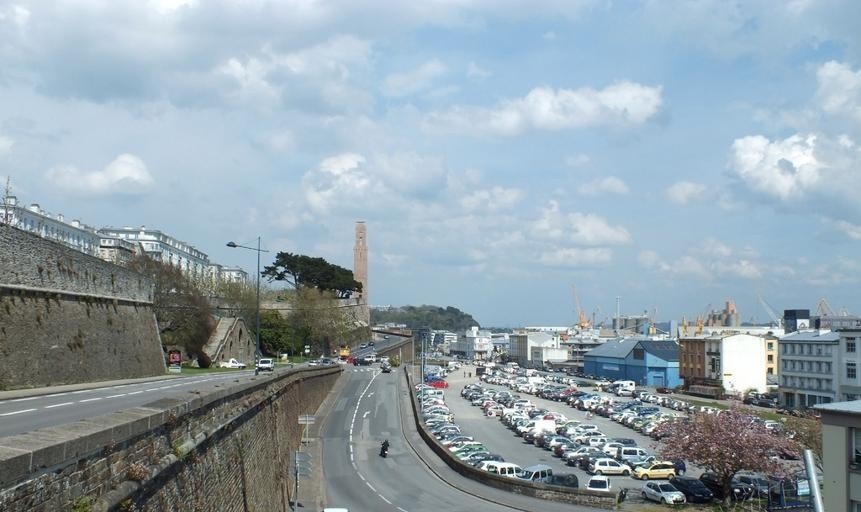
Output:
[379,441,391,458]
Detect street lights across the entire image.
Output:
[226,235,269,376]
[420,331,428,412]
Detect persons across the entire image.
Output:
[382,438,390,455]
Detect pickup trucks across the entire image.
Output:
[220,357,246,369]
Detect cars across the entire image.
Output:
[381,356,392,373]
[384,335,389,339]
[308,358,336,368]
[339,341,376,366]
[258,358,274,372]
